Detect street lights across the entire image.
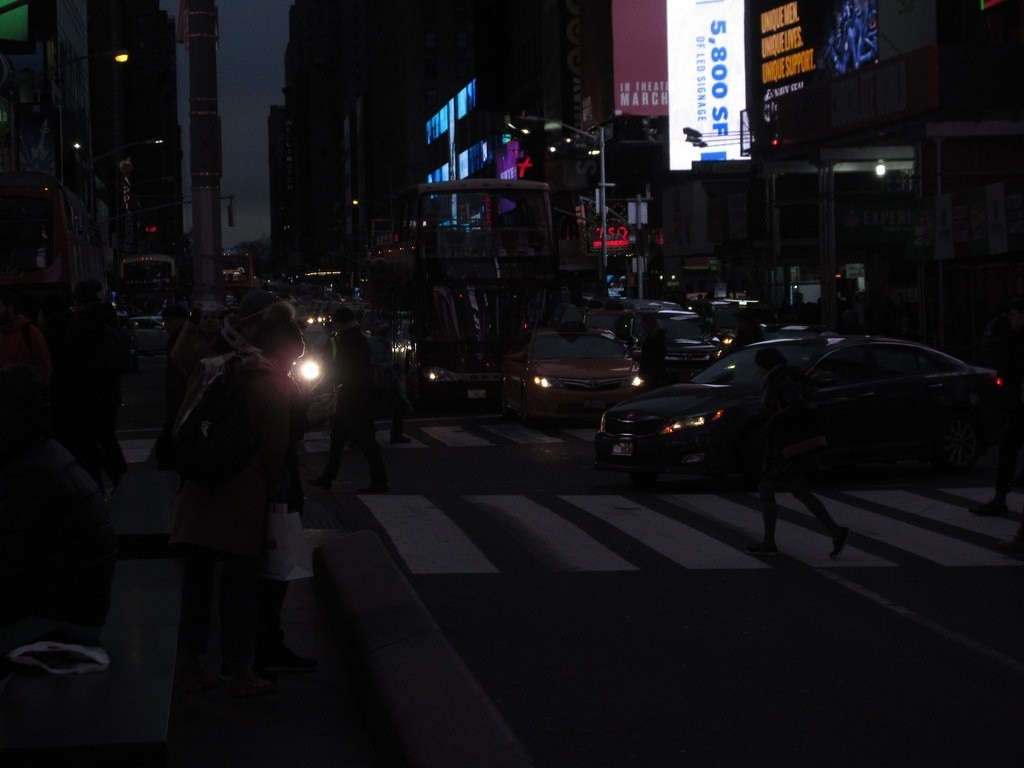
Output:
[516,114,616,286]
[89,137,163,225]
[15,48,130,173]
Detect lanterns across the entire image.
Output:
[144,224,156,233]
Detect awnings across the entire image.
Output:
[0,169,86,244]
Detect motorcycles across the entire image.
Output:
[293,317,339,419]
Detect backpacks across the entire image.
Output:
[171,351,268,482]
[81,314,131,375]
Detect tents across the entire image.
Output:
[0,259,106,296]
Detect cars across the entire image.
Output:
[279,281,380,320]
[596,334,1010,491]
[501,329,646,428]
[582,309,627,336]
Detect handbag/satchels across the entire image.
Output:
[266,504,314,582]
[778,400,826,459]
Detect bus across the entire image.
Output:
[120,255,175,319]
[393,177,581,413]
[221,248,254,307]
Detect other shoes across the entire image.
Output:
[356,482,387,495]
[251,644,320,671]
[231,672,278,693]
[745,543,777,556]
[992,530,1024,559]
[389,434,411,444]
[307,475,331,490]
[830,525,851,558]
[178,665,217,688]
[968,499,1008,517]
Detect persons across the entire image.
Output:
[691,293,707,320]
[635,309,676,395]
[0,279,415,693]
[968,295,1024,559]
[747,348,850,560]
[730,280,916,352]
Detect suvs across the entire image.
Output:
[616,301,774,384]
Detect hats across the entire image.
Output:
[756,346,787,372]
[332,308,354,325]
